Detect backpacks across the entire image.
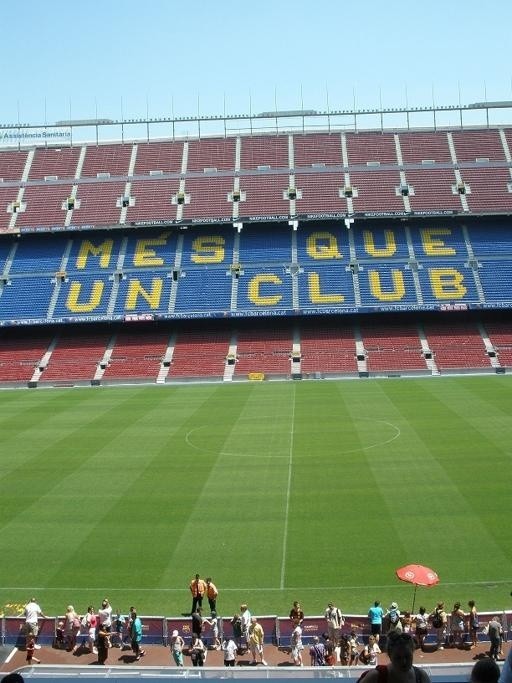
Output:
[433,608,444,629]
[389,608,400,625]
[416,615,428,633]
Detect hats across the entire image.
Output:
[172,629,179,638]
[376,652,392,666]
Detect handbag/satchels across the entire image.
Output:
[455,617,465,632]
[326,652,337,666]
[470,618,479,631]
[316,653,325,666]
[358,645,371,664]
[335,608,345,630]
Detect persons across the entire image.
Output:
[498,646,512,681]
[1,673,24,682]
[169,629,205,673]
[486,616,504,661]
[220,604,268,679]
[470,659,501,681]
[310,601,383,679]
[56,598,147,667]
[187,572,222,649]
[25,596,47,665]
[289,602,306,667]
[384,599,479,649]
[358,633,430,682]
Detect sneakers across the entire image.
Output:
[32,643,145,665]
[415,641,505,662]
[190,644,251,654]
[261,659,269,666]
[249,659,257,665]
